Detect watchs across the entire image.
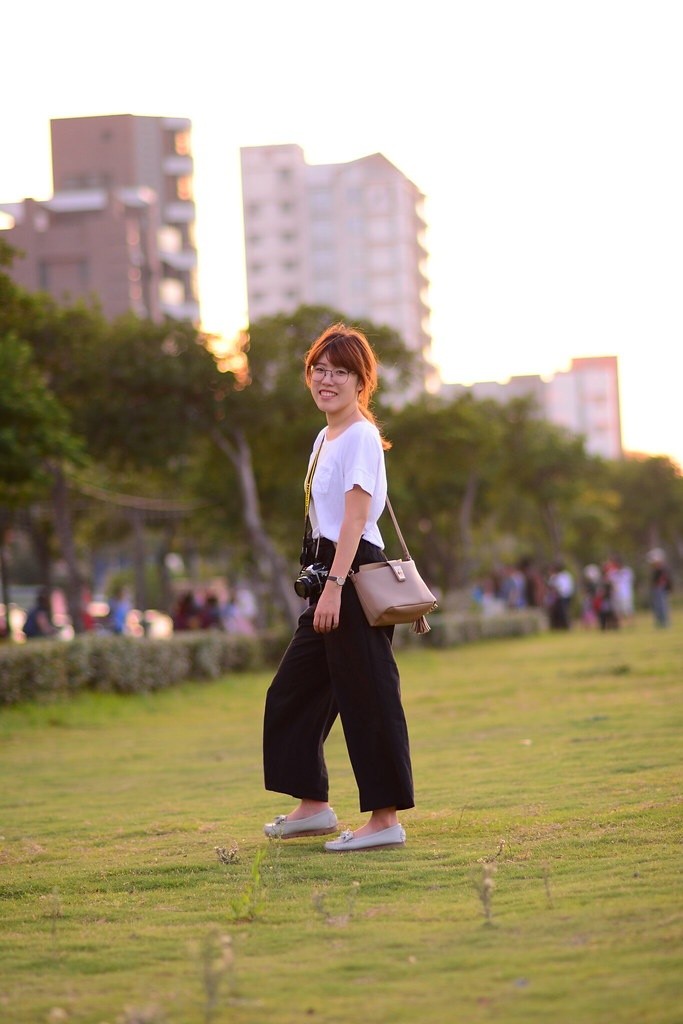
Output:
[328,576,345,586]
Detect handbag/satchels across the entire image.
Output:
[347,557,438,636]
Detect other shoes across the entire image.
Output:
[325,823,407,852]
[264,807,340,840]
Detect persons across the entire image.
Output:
[261,322,415,851]
[0,537,674,646]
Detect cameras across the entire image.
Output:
[293,563,329,600]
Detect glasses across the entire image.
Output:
[309,361,356,386]
